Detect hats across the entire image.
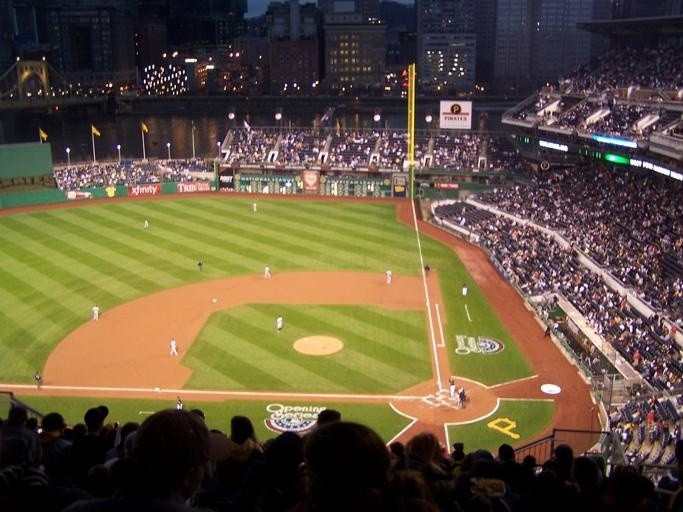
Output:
[83,405,109,426]
